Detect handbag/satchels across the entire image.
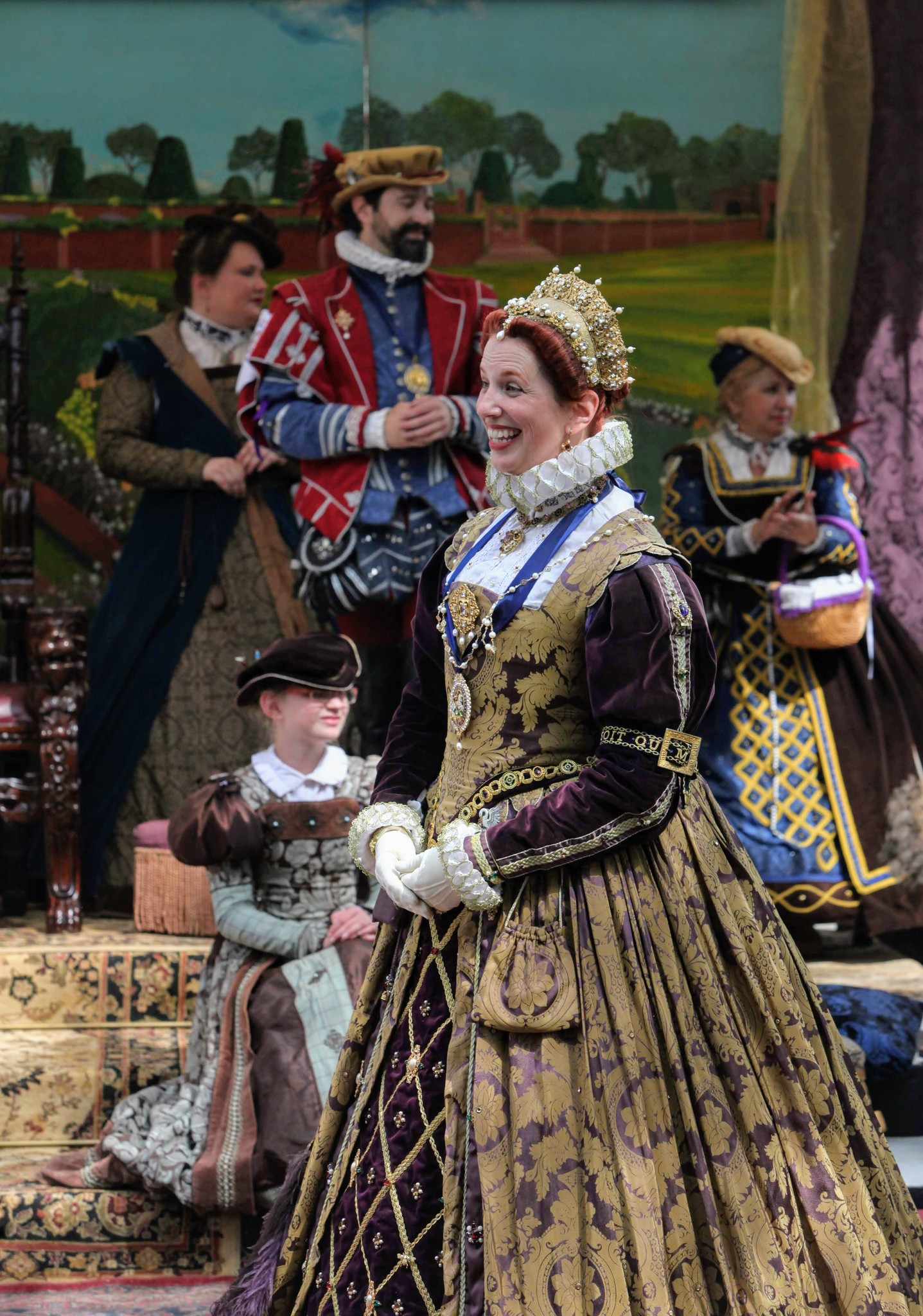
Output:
[470,920,582,1033]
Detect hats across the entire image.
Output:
[236,632,363,706]
[183,203,285,270]
[709,325,814,384]
[330,144,451,214]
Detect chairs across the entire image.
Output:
[0,236,124,933]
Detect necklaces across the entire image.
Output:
[436,503,655,751]
[499,474,610,553]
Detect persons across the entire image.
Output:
[208,264,923,1316]
[0,396,120,935]
[42,627,415,1222]
[660,323,923,956]
[75,200,389,929]
[235,146,506,780]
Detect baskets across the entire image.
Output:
[768,515,874,649]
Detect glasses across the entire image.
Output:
[281,686,359,705]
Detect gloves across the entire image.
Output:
[395,847,461,911]
[374,831,434,920]
